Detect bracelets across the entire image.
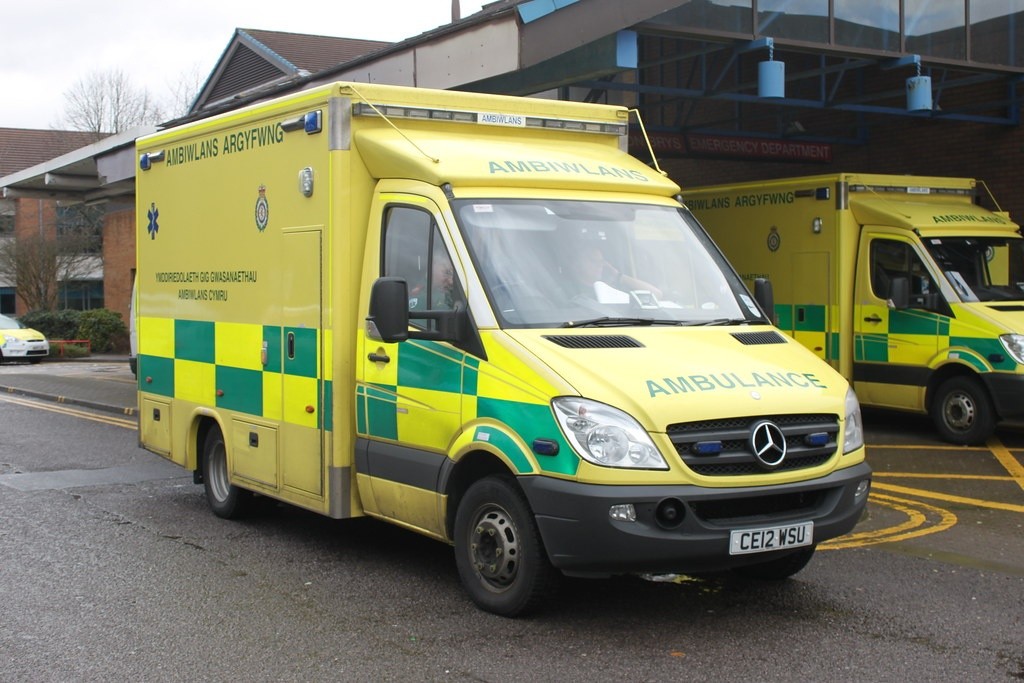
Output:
[617,272,624,286]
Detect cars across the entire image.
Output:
[0,312,48,365]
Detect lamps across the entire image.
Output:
[905,63,934,112]
[757,48,785,100]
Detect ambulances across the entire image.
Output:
[634,174,1024,448]
[133,80,871,621]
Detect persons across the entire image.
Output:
[556,245,662,311]
[409,245,460,330]
